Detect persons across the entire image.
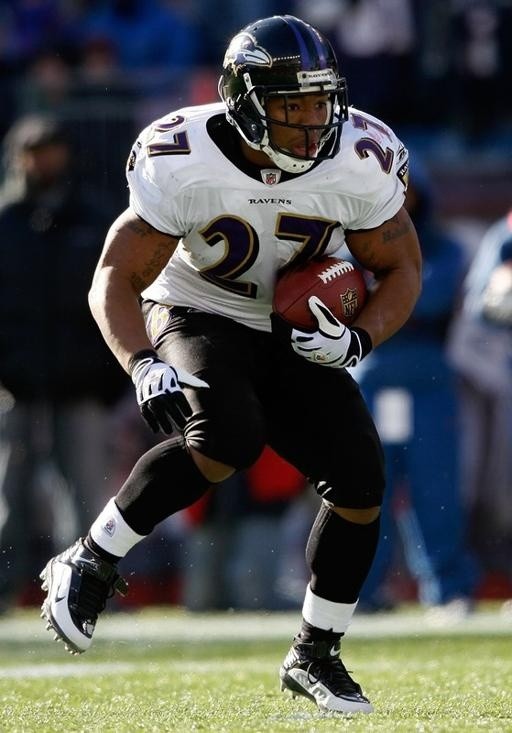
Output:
[2,0,511,140]
[2,112,165,614]
[38,10,424,717]
[440,199,511,628]
[346,145,493,623]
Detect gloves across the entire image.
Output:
[270,296,373,372]
[127,349,209,436]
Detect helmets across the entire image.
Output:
[218,15,350,175]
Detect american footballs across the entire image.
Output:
[272,255,366,334]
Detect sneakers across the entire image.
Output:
[40,536,114,654]
[279,634,375,712]
